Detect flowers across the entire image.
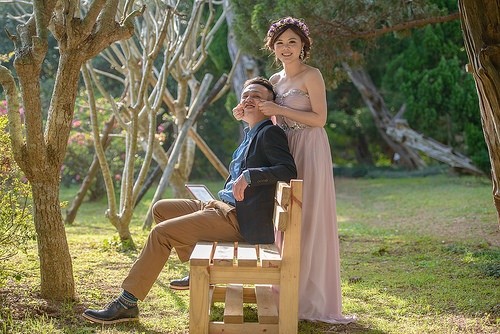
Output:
[268,17,309,37]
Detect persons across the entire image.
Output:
[82,76,297,324]
[232,17,354,324]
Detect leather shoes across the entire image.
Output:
[82,300,139,324]
[169,275,189,289]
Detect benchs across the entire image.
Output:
[189,178,303,334]
